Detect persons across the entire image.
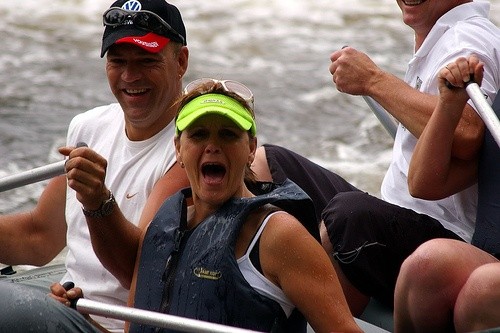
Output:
[247,0,500,318]
[48,81,366,333]
[391,55,500,333]
[0,0,191,333]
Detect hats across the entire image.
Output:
[101,0,187,58]
[174,93,256,140]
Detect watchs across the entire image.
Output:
[81,189,116,217]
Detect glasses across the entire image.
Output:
[103,7,185,45]
[182,77,256,118]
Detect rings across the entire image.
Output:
[446,63,451,69]
